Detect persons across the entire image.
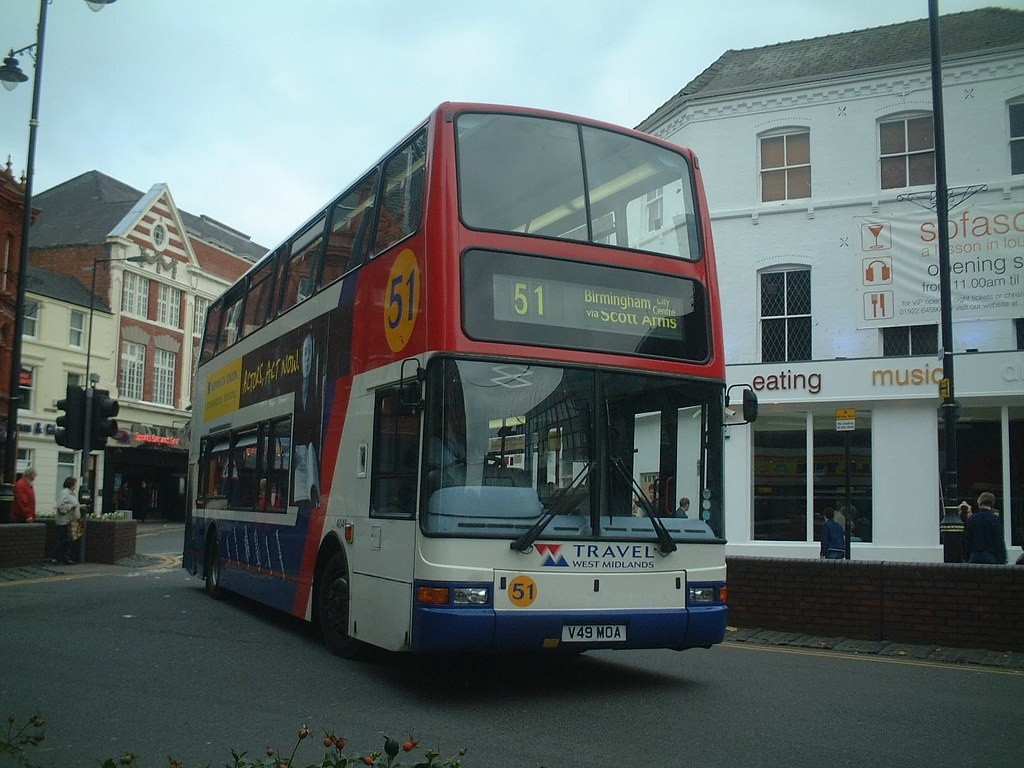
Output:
[50,477,88,564]
[136,481,152,523]
[257,478,286,510]
[9,468,37,524]
[959,492,1008,565]
[676,498,690,518]
[631,483,659,517]
[118,479,130,509]
[819,508,845,560]
[413,402,509,475]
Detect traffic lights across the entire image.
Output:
[55,383,86,450]
[92,389,120,441]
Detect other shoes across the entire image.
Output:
[64,558,76,565]
[47,558,58,564]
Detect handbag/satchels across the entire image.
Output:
[67,507,83,541]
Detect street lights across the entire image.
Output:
[1,1,116,522]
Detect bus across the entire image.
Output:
[180,100,755,655]
